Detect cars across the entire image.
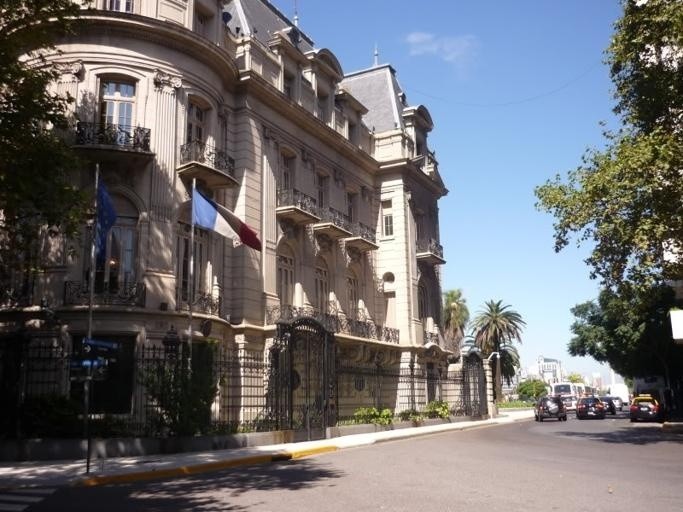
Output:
[629,393,664,422]
[575,396,605,420]
[598,396,615,416]
[609,396,623,411]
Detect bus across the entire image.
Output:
[546,381,599,412]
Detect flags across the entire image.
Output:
[189,187,262,254]
[96,175,116,259]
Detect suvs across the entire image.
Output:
[531,394,567,422]
